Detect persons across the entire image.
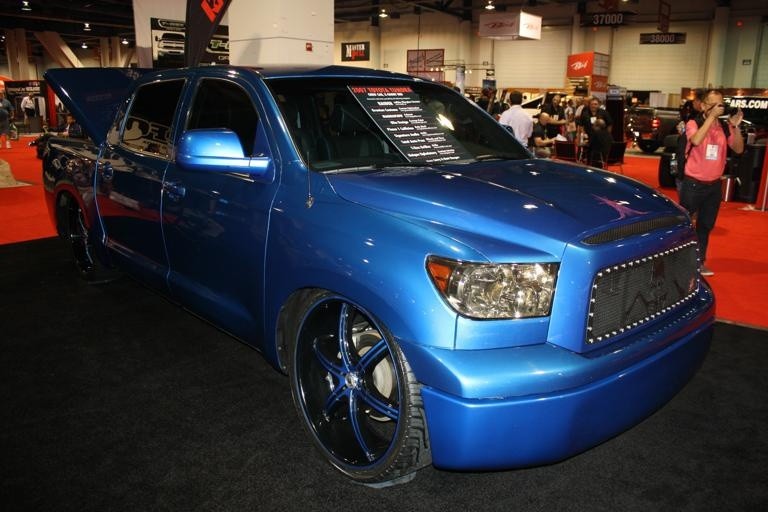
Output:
[676,89,745,276]
[61,114,81,136]
[20,91,35,134]
[468,78,613,166]
[0,90,16,150]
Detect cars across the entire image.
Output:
[517,91,572,123]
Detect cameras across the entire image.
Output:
[717,103,738,116]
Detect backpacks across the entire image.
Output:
[668,116,732,181]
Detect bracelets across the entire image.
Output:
[731,124,740,127]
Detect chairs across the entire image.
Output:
[320,94,383,163]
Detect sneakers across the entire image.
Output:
[697,265,715,277]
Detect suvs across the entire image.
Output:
[26,53,716,493]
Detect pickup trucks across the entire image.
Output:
[624,104,756,154]
